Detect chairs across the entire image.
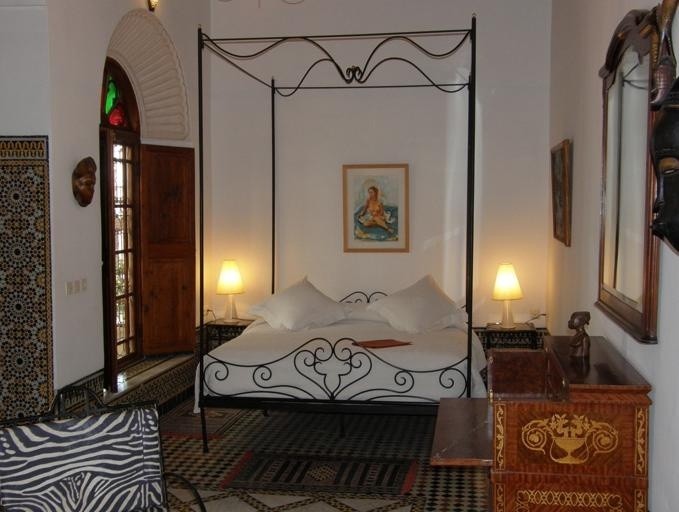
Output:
[0,386,206,512]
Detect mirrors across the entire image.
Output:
[594,8,659,346]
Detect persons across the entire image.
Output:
[357,186,396,235]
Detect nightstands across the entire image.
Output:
[484,322,537,349]
[205,319,255,353]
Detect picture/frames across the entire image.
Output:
[550,139,570,247]
[341,163,409,253]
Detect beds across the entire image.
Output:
[196,16,478,454]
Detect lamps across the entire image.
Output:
[215,259,246,323]
[491,263,524,329]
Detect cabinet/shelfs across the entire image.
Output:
[428,335,653,511]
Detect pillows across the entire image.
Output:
[247,272,469,336]
